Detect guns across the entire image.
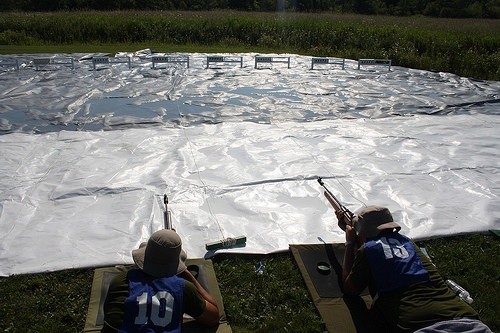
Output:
[316,178,357,233]
[163,194,174,235]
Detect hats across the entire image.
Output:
[132,229,188,278]
[356,206,401,239]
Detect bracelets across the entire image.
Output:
[345,241,353,246]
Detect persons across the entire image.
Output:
[101,229,219,333]
[335,206,481,333]
